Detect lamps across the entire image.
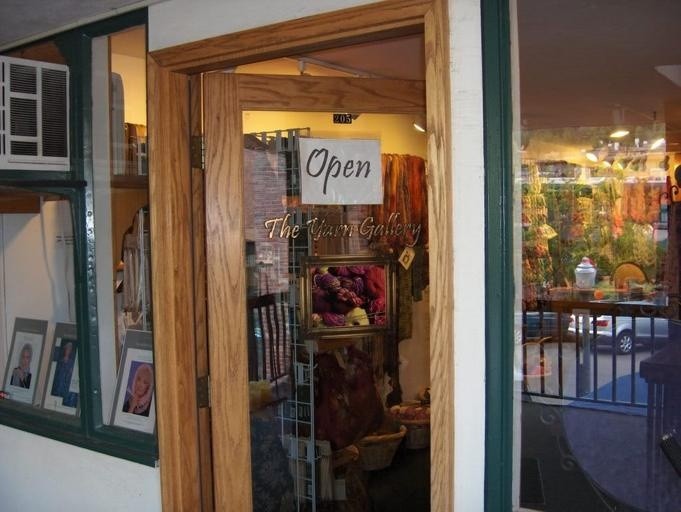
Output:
[583,145,670,172]
[411,117,426,133]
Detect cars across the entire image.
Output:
[524,300,572,342]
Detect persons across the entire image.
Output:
[60,342,73,363]
[123,363,154,417]
[9,343,34,389]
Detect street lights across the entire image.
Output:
[256,236,275,289]
[573,255,598,396]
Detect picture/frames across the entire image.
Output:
[40,321,82,419]
[109,328,157,438]
[1,316,49,407]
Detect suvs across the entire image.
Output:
[569,299,670,353]
